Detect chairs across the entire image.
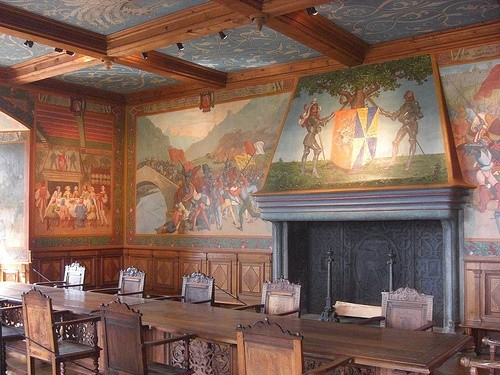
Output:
[0,260,500,375]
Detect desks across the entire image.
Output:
[0,281,474,375]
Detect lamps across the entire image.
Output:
[177,43,185,51]
[218,31,227,40]
[306,6,318,16]
[54,48,75,56]
[23,40,34,48]
[141,53,148,59]
